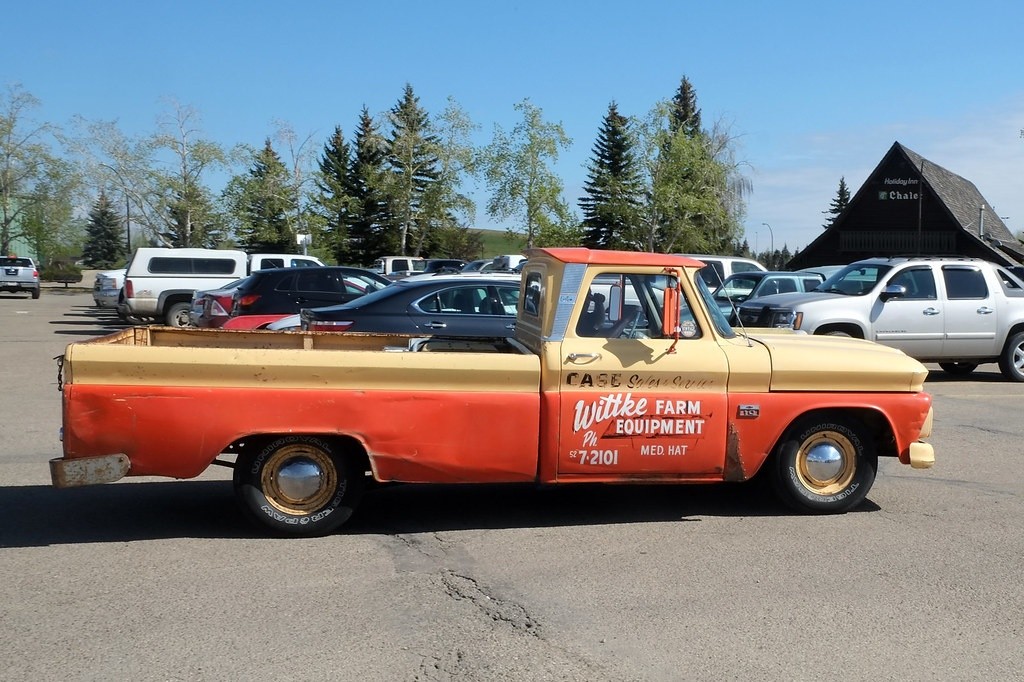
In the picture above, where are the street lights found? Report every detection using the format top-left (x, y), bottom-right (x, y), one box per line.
top-left (762, 223), bottom-right (773, 262)
top-left (98, 162), bottom-right (131, 254)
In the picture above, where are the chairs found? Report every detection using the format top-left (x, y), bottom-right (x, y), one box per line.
top-left (442, 293), bottom-right (498, 314)
top-left (525, 294), bottom-right (538, 316)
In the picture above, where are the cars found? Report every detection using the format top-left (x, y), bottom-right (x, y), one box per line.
top-left (300, 278), bottom-right (616, 338)
top-left (188, 275), bottom-right (365, 328)
top-left (93, 267), bottom-right (153, 326)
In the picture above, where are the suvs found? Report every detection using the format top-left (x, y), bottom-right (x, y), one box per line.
top-left (232, 265), bottom-right (391, 318)
top-left (0, 256), bottom-right (42, 299)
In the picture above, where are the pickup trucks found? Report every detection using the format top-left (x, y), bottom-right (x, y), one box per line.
top-left (729, 254), bottom-right (1024, 384)
top-left (48, 247), bottom-right (936, 539)
top-left (222, 253), bottom-right (879, 339)
top-left (117, 248), bottom-right (326, 328)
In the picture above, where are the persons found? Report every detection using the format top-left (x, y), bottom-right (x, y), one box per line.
top-left (578, 288), bottom-right (605, 335)
